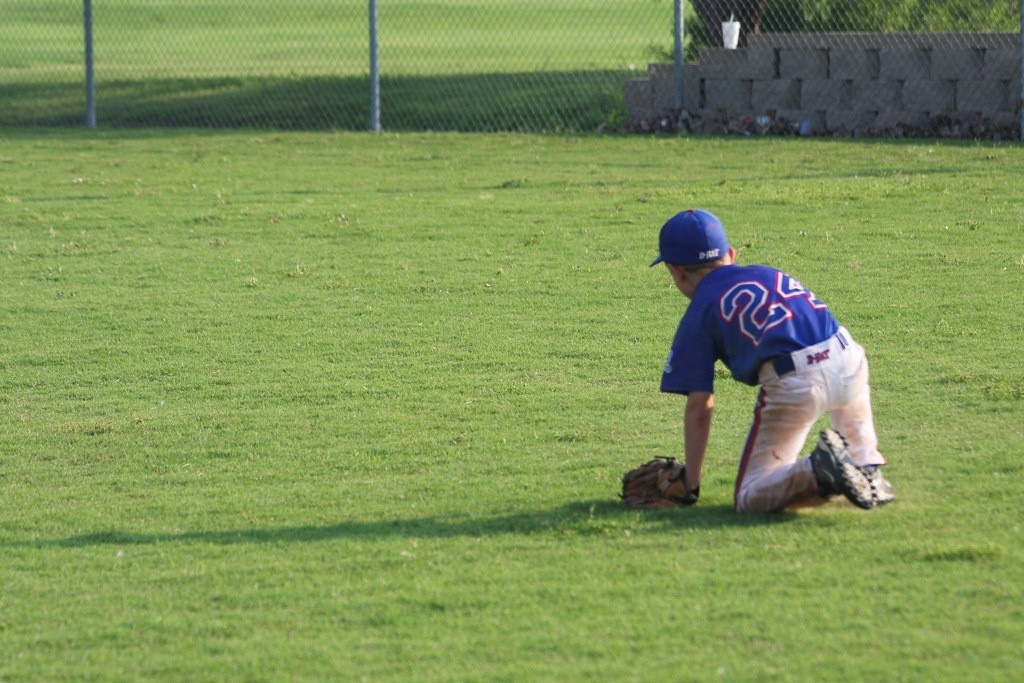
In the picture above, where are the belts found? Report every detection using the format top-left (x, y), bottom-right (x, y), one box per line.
top-left (772, 333), bottom-right (848, 377)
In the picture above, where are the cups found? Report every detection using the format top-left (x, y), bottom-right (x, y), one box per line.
top-left (723, 22), bottom-right (740, 49)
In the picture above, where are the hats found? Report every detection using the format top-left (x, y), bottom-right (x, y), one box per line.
top-left (649, 210), bottom-right (728, 267)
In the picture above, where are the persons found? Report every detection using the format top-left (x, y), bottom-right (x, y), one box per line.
top-left (650, 209), bottom-right (894, 516)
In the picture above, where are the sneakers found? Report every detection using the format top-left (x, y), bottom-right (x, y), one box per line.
top-left (811, 428), bottom-right (878, 510)
top-left (857, 464), bottom-right (894, 503)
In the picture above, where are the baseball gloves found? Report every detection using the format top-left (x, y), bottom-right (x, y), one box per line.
top-left (618, 454), bottom-right (700, 510)
